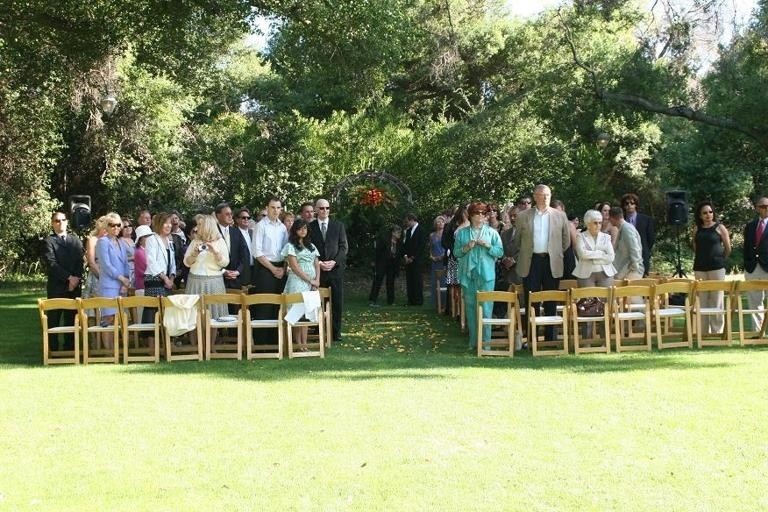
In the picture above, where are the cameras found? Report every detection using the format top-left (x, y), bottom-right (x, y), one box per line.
top-left (198, 243), bottom-right (209, 252)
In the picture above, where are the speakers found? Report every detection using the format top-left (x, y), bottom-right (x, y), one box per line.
top-left (68, 194), bottom-right (91, 228)
top-left (665, 190), bottom-right (689, 227)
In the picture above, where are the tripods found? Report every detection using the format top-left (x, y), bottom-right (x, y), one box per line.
top-left (670, 228), bottom-right (687, 278)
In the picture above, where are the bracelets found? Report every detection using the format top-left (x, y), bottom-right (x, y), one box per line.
top-left (404, 255), bottom-right (407, 257)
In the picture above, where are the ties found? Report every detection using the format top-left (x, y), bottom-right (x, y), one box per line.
top-left (61, 235), bottom-right (65, 245)
top-left (321, 223), bottom-right (327, 241)
top-left (755, 220), bottom-right (765, 246)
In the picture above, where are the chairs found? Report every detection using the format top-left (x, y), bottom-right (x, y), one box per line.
top-left (38, 284), bottom-right (333, 366)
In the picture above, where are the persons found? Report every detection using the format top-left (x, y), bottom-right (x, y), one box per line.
top-left (428, 183), bottom-right (655, 355)
top-left (692, 203), bottom-right (731, 334)
top-left (368, 226), bottom-right (404, 306)
top-left (401, 214), bottom-right (430, 306)
top-left (44, 198), bottom-right (349, 352)
top-left (744, 197), bottom-right (768, 338)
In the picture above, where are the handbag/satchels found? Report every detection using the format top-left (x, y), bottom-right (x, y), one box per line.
top-left (576, 296), bottom-right (605, 317)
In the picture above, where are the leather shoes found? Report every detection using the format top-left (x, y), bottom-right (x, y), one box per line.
top-left (333, 337), bottom-right (342, 341)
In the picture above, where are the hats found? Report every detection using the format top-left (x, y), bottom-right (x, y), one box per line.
top-left (134, 225), bottom-right (156, 244)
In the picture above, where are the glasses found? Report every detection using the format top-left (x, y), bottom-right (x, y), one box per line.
top-left (594, 222), bottom-right (602, 224)
top-left (108, 223), bottom-right (121, 227)
top-left (225, 212), bottom-right (233, 216)
top-left (238, 216), bottom-right (251, 219)
top-left (703, 210), bottom-right (713, 214)
top-left (55, 219), bottom-right (66, 223)
top-left (759, 205), bottom-right (768, 208)
top-left (488, 209), bottom-right (497, 212)
top-left (192, 230), bottom-right (197, 233)
top-left (124, 225), bottom-right (133, 228)
top-left (475, 211), bottom-right (487, 215)
top-left (625, 201), bottom-right (635, 205)
top-left (520, 201), bottom-right (532, 205)
top-left (316, 207), bottom-right (329, 210)
